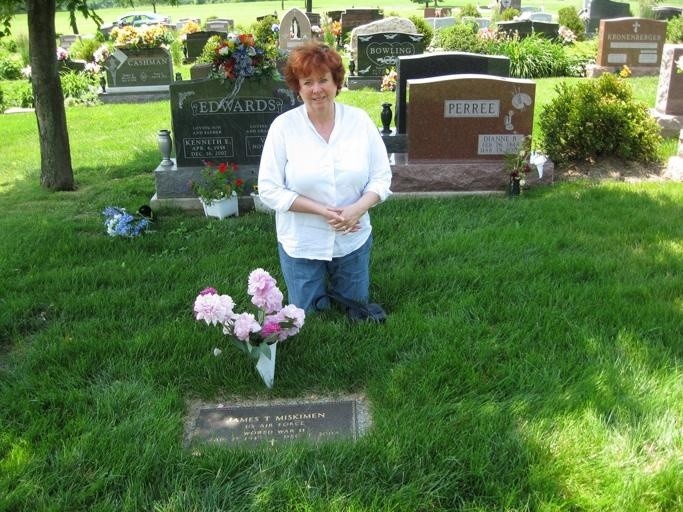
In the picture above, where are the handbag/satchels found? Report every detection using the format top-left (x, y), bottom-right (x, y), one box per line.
top-left (312, 294), bottom-right (389, 322)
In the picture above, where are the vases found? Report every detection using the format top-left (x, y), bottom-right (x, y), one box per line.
top-left (156, 130), bottom-right (174, 167)
top-left (250, 192), bottom-right (273, 215)
top-left (245, 338), bottom-right (278, 389)
top-left (510, 176), bottom-right (521, 196)
top-left (196, 191), bottom-right (239, 221)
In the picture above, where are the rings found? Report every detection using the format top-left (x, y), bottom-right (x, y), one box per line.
top-left (342, 225), bottom-right (346, 230)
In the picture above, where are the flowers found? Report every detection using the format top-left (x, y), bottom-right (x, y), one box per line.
top-left (379, 67), bottom-right (397, 92)
top-left (102, 207), bottom-right (148, 237)
top-left (619, 64), bottom-right (631, 78)
top-left (193, 266), bottom-right (305, 364)
top-left (187, 158), bottom-right (243, 207)
top-left (201, 35), bottom-right (289, 100)
top-left (109, 24), bottom-right (174, 50)
top-left (502, 134), bottom-right (534, 186)
top-left (251, 180), bottom-right (258, 195)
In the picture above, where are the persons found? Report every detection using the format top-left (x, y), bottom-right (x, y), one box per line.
top-left (256, 43), bottom-right (392, 317)
